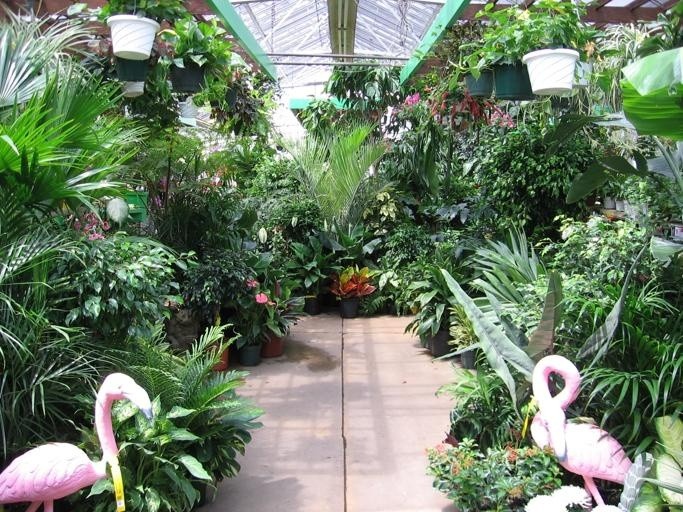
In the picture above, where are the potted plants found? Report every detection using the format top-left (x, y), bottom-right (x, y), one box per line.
top-left (280, 57), bottom-right (405, 319)
top-left (2, 1), bottom-right (292, 512)
top-left (394, 0), bottom-right (682, 512)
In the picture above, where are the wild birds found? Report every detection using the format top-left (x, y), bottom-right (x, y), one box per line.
top-left (0, 371), bottom-right (151, 512)
top-left (529, 353), bottom-right (633, 510)
